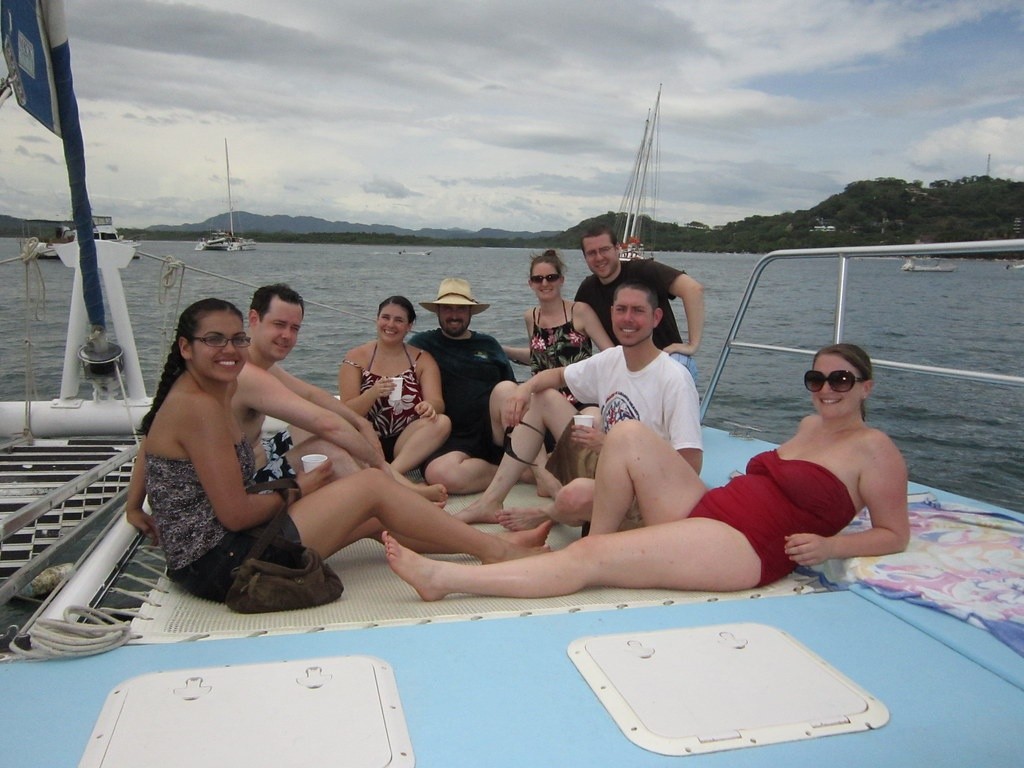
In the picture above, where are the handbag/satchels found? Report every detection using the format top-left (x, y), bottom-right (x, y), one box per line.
top-left (224, 547), bottom-right (344, 614)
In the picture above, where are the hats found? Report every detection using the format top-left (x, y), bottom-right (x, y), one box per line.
top-left (418, 278), bottom-right (490, 315)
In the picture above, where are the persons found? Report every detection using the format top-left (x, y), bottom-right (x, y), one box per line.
top-left (229, 284), bottom-right (449, 510)
top-left (126, 298), bottom-right (554, 603)
top-left (380, 345), bottom-right (911, 600)
top-left (500, 252), bottom-right (616, 454)
top-left (406, 278), bottom-right (565, 500)
top-left (451, 277), bottom-right (703, 533)
top-left (574, 223), bottom-right (705, 383)
top-left (339, 295), bottom-right (451, 474)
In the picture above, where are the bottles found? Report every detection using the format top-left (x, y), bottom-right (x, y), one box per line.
top-left (23, 563), bottom-right (75, 597)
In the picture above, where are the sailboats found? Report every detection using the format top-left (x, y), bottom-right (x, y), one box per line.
top-left (610, 84), bottom-right (663, 263)
top-left (200, 137), bottom-right (257, 250)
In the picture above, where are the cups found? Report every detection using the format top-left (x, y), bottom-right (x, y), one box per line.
top-left (389, 378), bottom-right (403, 400)
top-left (301, 454), bottom-right (327, 473)
top-left (574, 415), bottom-right (594, 433)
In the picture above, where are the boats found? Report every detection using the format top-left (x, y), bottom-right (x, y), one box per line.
top-left (901, 260), bottom-right (958, 272)
top-left (1005, 263), bottom-right (1023, 269)
top-left (0, 232), bottom-right (1023, 766)
top-left (396, 250), bottom-right (432, 255)
top-left (31, 216), bottom-right (142, 260)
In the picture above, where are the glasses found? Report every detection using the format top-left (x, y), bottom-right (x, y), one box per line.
top-left (530, 274), bottom-right (560, 283)
top-left (189, 335), bottom-right (251, 348)
top-left (804, 370), bottom-right (865, 392)
top-left (503, 420), bottom-right (547, 467)
